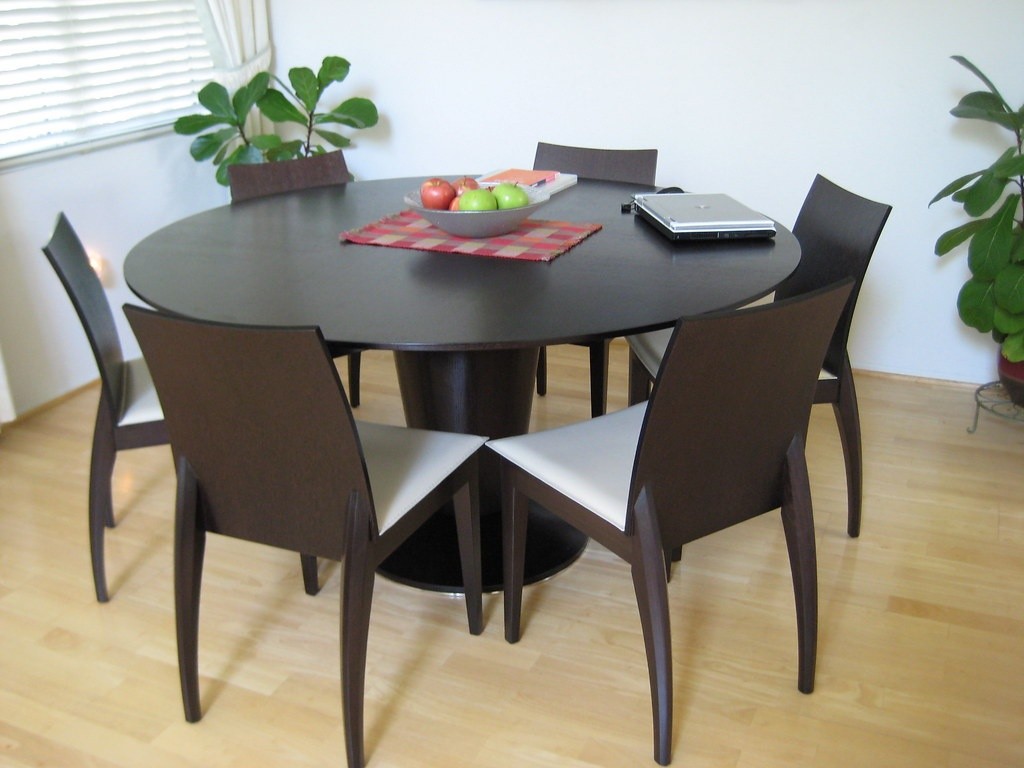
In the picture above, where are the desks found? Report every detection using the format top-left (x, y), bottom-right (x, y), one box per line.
top-left (123, 174), bottom-right (802, 593)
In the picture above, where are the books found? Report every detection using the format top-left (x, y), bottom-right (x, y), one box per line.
top-left (477, 171), bottom-right (578, 196)
top-left (480, 167), bottom-right (559, 188)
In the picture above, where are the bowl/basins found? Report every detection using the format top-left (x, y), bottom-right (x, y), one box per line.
top-left (405, 181), bottom-right (551, 238)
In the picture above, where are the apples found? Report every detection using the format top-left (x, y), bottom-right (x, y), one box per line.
top-left (421, 178), bottom-right (529, 210)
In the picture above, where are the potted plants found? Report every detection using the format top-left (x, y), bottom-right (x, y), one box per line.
top-left (928, 52), bottom-right (1024, 407)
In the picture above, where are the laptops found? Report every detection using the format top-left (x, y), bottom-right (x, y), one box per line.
top-left (635, 192), bottom-right (776, 242)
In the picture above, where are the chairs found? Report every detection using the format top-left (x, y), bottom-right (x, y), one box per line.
top-left (122, 302), bottom-right (490, 768)
top-left (623, 172), bottom-right (893, 538)
top-left (532, 141), bottom-right (658, 418)
top-left (484, 275), bottom-right (860, 766)
top-left (41, 210), bottom-right (319, 603)
top-left (227, 149), bottom-right (361, 409)
